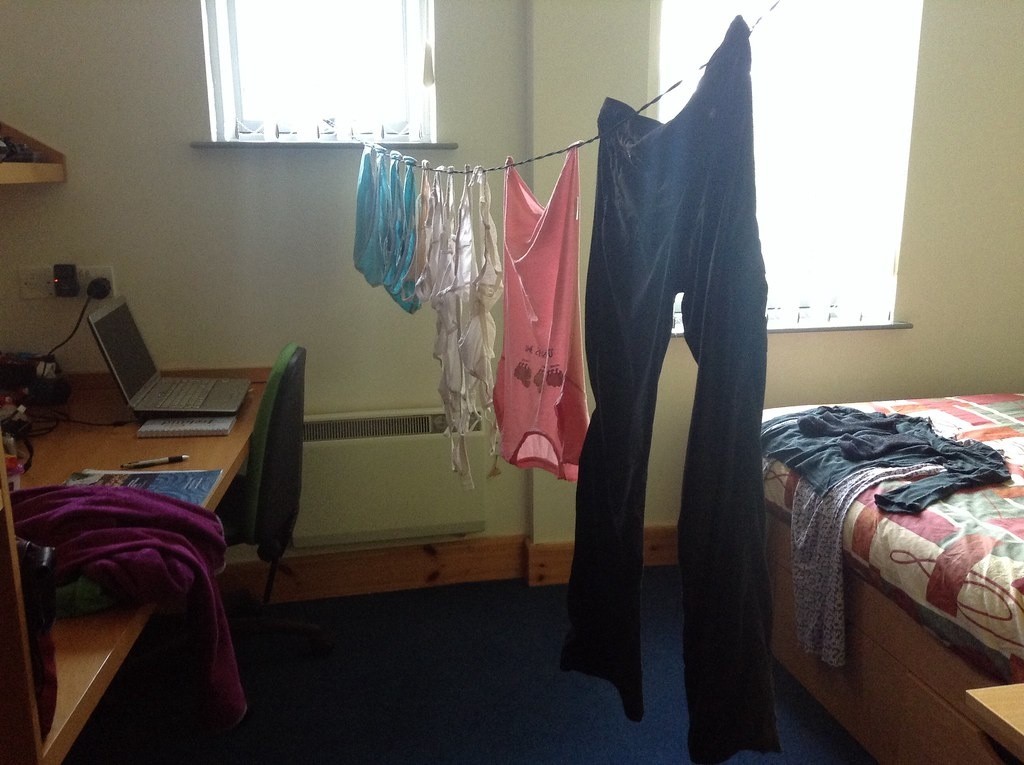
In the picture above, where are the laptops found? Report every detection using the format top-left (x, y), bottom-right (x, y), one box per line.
top-left (86, 294), bottom-right (251, 416)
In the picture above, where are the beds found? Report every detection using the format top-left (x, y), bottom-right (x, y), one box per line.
top-left (759, 393), bottom-right (1024, 765)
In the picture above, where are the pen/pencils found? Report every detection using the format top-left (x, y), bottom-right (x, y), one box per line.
top-left (121, 454), bottom-right (189, 468)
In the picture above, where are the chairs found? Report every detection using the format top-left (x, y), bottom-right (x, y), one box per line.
top-left (209, 344), bottom-right (307, 618)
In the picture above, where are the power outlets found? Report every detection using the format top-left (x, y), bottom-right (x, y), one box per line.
top-left (18, 263), bottom-right (117, 303)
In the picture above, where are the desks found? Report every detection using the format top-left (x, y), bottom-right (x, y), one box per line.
top-left (0, 366), bottom-right (272, 765)
